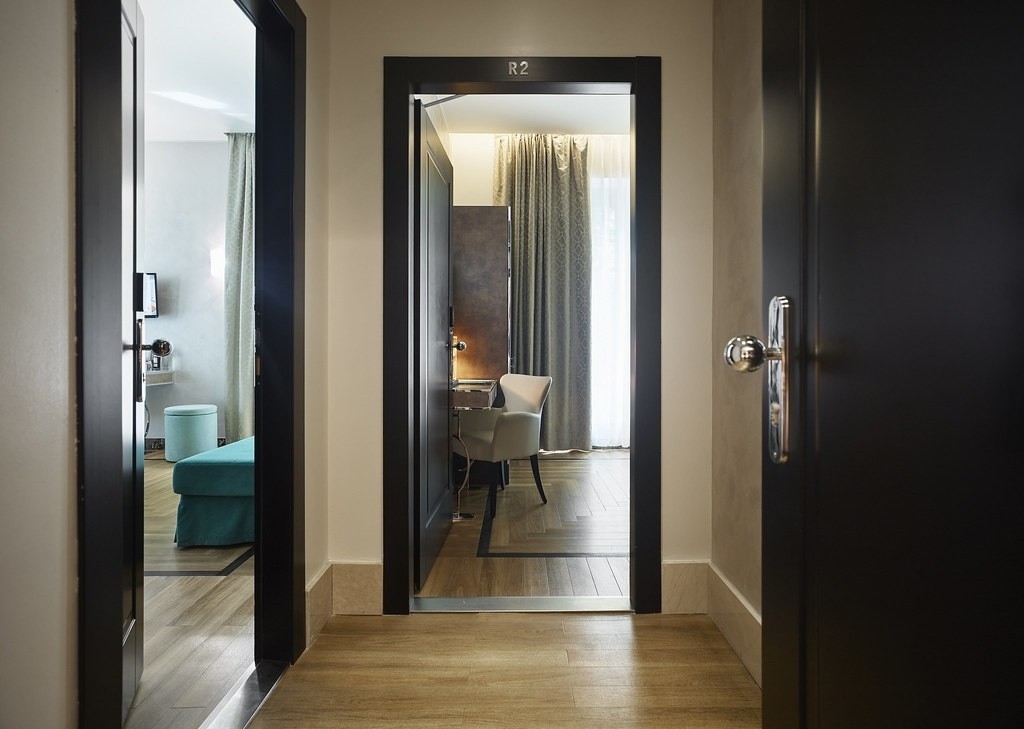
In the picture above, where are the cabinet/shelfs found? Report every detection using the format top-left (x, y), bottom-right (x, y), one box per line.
top-left (455, 205), bottom-right (512, 392)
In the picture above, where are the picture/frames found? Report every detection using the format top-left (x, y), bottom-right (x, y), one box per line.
top-left (142, 273), bottom-right (158, 318)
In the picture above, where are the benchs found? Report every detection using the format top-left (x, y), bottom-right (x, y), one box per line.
top-left (174, 435), bottom-right (255, 548)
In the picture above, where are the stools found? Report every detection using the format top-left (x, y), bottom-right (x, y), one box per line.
top-left (164, 404), bottom-right (217, 462)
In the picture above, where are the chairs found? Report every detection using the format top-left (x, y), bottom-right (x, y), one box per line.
top-left (451, 374), bottom-right (552, 518)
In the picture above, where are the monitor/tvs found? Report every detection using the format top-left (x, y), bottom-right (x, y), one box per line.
top-left (136, 273), bottom-right (158, 319)
